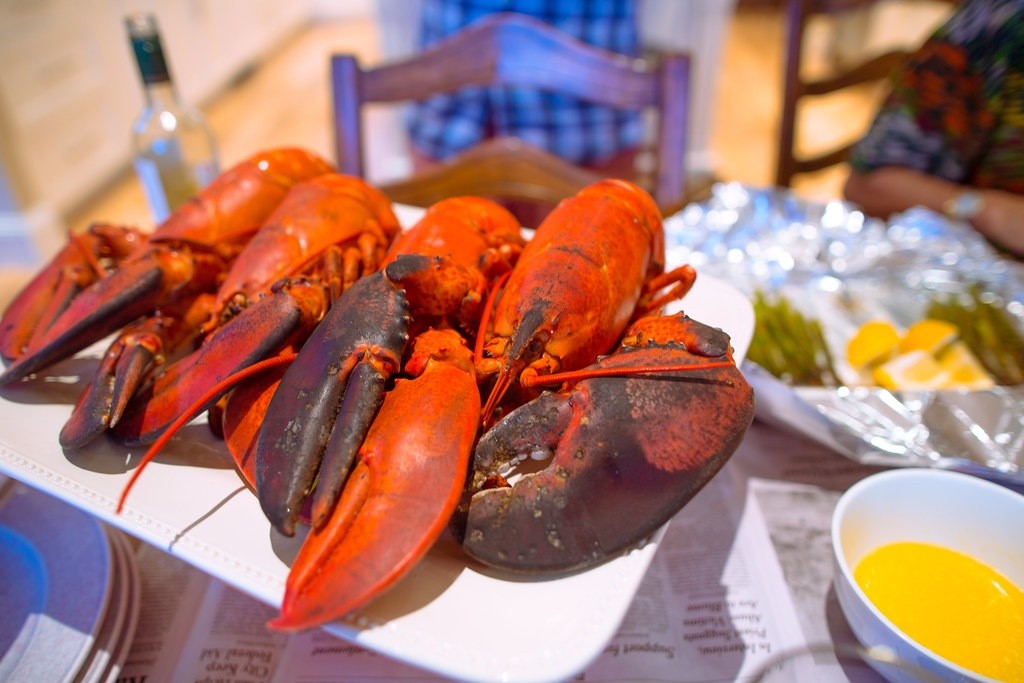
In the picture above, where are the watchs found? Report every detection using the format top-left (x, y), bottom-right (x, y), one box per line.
top-left (944, 185), bottom-right (985, 223)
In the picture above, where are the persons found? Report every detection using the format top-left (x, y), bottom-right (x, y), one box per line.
top-left (843, 1), bottom-right (1024, 256)
top-left (408, 1), bottom-right (640, 229)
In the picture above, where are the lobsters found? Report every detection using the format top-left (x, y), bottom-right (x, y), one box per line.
top-left (0, 147), bottom-right (757, 634)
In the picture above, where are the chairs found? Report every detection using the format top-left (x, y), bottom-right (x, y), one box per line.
top-left (777, 0), bottom-right (973, 189)
top-left (331, 11), bottom-right (690, 224)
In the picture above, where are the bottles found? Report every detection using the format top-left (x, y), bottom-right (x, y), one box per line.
top-left (124, 12), bottom-right (223, 222)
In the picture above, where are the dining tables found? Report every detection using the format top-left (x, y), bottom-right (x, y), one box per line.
top-left (0, 202), bottom-right (1024, 682)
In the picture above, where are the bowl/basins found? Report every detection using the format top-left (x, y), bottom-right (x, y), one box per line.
top-left (830, 468), bottom-right (1024, 683)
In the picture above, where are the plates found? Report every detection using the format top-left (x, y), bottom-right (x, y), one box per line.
top-left (0, 485), bottom-right (142, 683)
top-left (0, 198), bottom-right (755, 683)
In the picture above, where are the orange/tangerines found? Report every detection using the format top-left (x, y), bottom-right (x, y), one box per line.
top-left (849, 319), bottom-right (996, 394)
